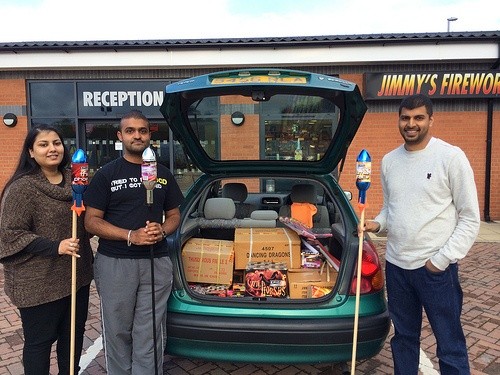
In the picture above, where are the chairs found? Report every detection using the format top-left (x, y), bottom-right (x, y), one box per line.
top-left (279, 185), bottom-right (330, 227)
top-left (220, 184), bottom-right (255, 216)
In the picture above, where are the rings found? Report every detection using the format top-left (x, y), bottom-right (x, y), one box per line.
top-left (70, 243), bottom-right (73, 246)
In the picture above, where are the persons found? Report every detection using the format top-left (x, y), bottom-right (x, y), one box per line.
top-left (0, 124), bottom-right (94, 375)
top-left (83, 112), bottom-right (185, 375)
top-left (357, 93), bottom-right (480, 374)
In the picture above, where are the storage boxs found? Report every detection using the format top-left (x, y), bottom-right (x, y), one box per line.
top-left (287, 266), bottom-right (339, 299)
top-left (183, 238), bottom-right (233, 286)
top-left (234, 227), bottom-right (302, 271)
top-left (244, 261), bottom-right (289, 298)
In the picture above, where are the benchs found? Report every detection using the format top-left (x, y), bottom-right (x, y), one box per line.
top-left (200, 198), bottom-right (278, 238)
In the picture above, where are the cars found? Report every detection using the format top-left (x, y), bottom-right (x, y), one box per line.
top-left (159, 68), bottom-right (392, 365)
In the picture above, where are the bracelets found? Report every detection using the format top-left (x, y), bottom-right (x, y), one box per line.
top-left (127, 230), bottom-right (131, 246)
top-left (163, 231), bottom-right (167, 236)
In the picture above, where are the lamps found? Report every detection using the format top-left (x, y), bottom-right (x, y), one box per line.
top-left (446, 17), bottom-right (458, 31)
